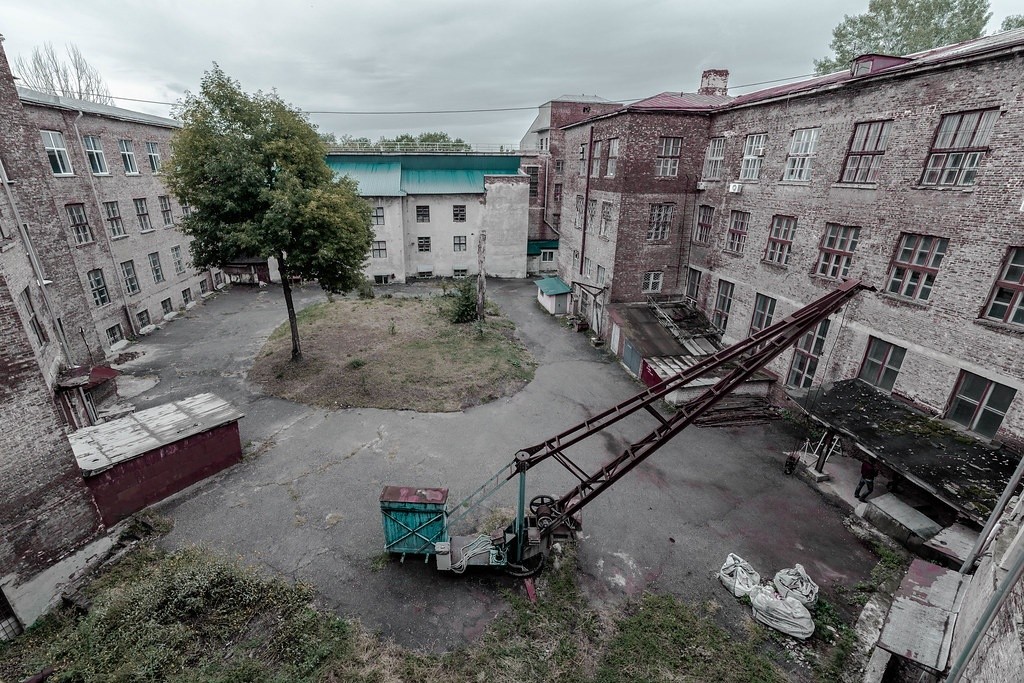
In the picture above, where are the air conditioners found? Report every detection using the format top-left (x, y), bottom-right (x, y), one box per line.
top-left (579, 146), bottom-right (584, 152)
top-left (579, 154), bottom-right (584, 158)
top-left (729, 183), bottom-right (743, 193)
top-left (697, 181), bottom-right (707, 190)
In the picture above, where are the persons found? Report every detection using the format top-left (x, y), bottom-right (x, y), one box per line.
top-left (855, 455), bottom-right (878, 503)
top-left (886, 471), bottom-right (905, 492)
